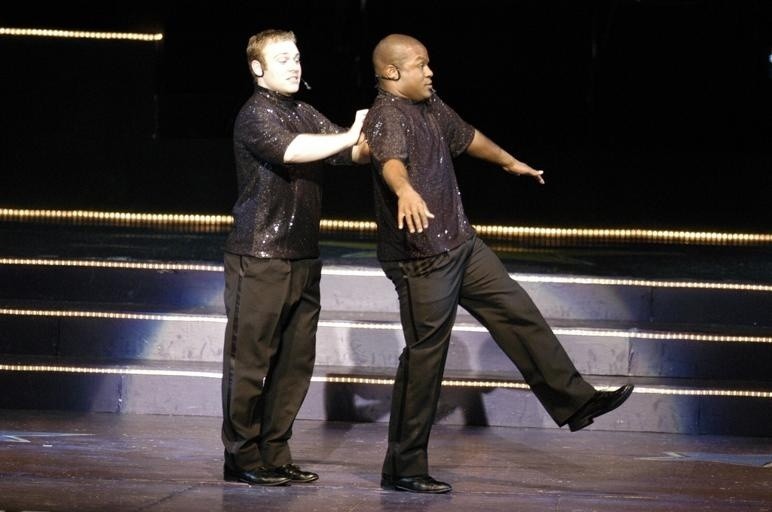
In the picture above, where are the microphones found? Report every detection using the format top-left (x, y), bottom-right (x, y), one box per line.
top-left (301, 78), bottom-right (312, 90)
top-left (432, 88), bottom-right (436, 93)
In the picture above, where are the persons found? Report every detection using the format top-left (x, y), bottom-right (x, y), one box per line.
top-left (361, 32), bottom-right (634, 493)
top-left (218, 28), bottom-right (377, 489)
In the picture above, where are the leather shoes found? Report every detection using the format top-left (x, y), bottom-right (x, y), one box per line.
top-left (280, 464), bottom-right (321, 483)
top-left (380, 472), bottom-right (455, 496)
top-left (567, 382), bottom-right (636, 436)
top-left (221, 465), bottom-right (294, 488)
